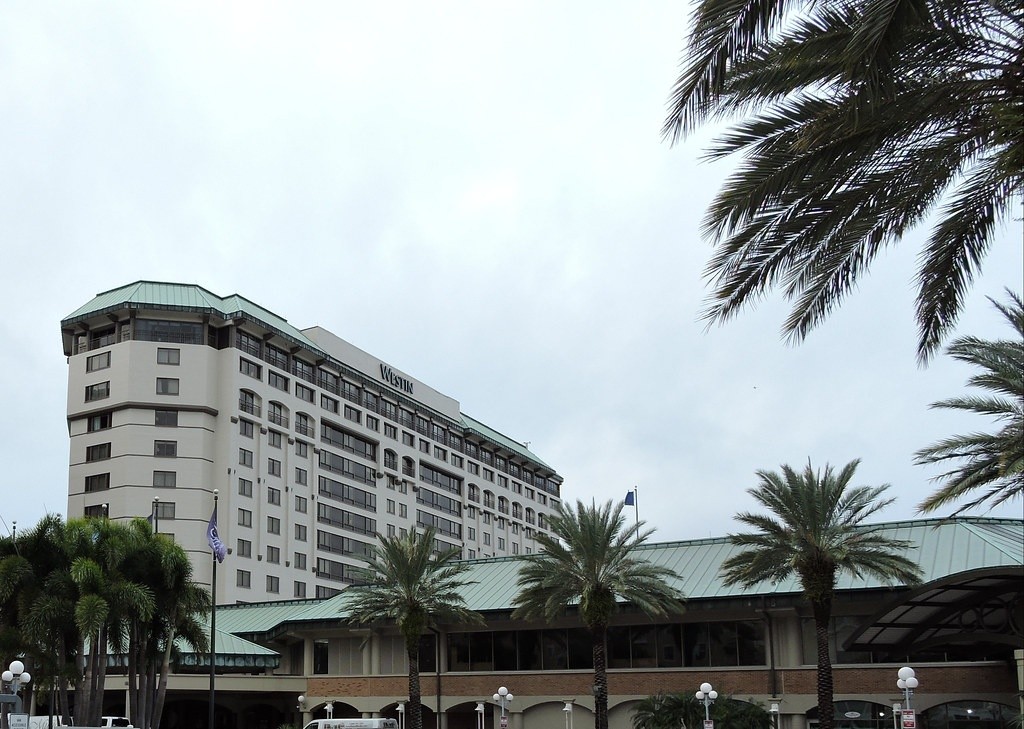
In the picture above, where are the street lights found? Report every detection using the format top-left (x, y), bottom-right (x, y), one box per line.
top-left (0, 660), bottom-right (32, 695)
top-left (896, 666), bottom-right (919, 710)
top-left (492, 686), bottom-right (514, 716)
top-left (695, 682), bottom-right (718, 720)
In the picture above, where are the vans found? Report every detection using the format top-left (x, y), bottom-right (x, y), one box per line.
top-left (69, 716), bottom-right (134, 729)
top-left (302, 717), bottom-right (399, 729)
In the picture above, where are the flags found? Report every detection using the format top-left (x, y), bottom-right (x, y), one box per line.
top-left (625, 491), bottom-right (634, 506)
top-left (206, 508), bottom-right (228, 564)
